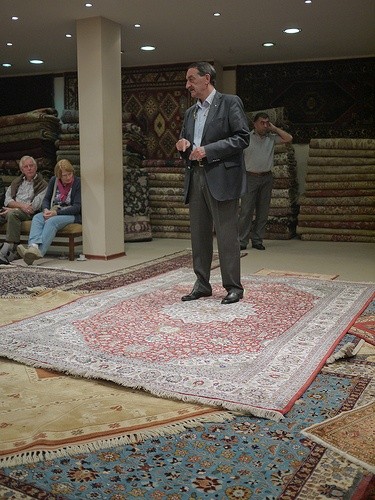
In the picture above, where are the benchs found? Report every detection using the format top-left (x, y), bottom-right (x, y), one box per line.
top-left (0, 183), bottom-right (82, 261)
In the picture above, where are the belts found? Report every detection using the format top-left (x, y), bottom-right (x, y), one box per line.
top-left (247, 170), bottom-right (272, 176)
top-left (192, 160), bottom-right (204, 167)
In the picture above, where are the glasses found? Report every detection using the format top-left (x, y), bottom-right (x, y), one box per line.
top-left (260, 121), bottom-right (269, 125)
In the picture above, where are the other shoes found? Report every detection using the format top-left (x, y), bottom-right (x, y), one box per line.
top-left (0, 242), bottom-right (15, 264)
top-left (16, 245), bottom-right (39, 259)
top-left (23, 252), bottom-right (36, 265)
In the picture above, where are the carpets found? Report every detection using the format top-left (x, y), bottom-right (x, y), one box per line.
top-left (1, 266), bottom-right (375, 423)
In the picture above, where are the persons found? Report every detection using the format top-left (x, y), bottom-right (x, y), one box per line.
top-left (0, 155), bottom-right (49, 264)
top-left (241, 113), bottom-right (293, 251)
top-left (23, 159), bottom-right (81, 265)
top-left (175, 62), bottom-right (249, 306)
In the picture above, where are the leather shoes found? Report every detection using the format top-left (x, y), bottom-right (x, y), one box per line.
top-left (221, 291), bottom-right (243, 304)
top-left (252, 244), bottom-right (265, 250)
top-left (181, 288), bottom-right (212, 301)
top-left (240, 246), bottom-right (247, 250)
